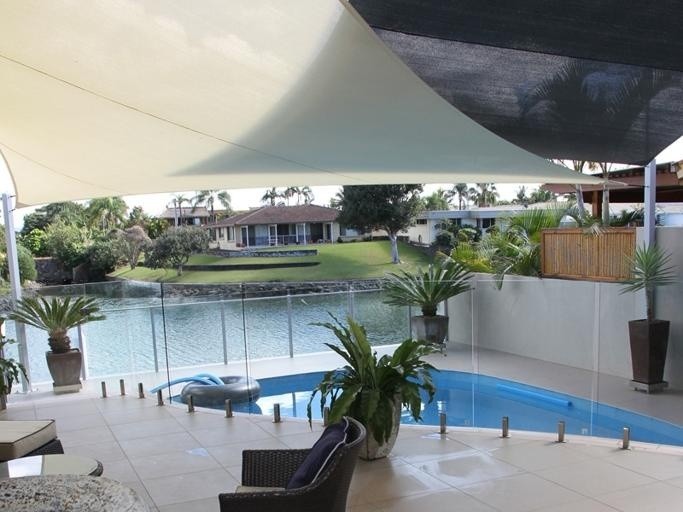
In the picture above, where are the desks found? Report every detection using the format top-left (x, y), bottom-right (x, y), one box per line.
top-left (0, 454), bottom-right (103, 483)
top-left (0, 474), bottom-right (151, 512)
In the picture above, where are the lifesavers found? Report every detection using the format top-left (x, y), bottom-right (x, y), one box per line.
top-left (181, 377), bottom-right (260, 405)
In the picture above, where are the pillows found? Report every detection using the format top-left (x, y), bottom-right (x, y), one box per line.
top-left (285, 416), bottom-right (350, 488)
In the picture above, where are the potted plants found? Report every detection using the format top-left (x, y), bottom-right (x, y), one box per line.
top-left (380, 260), bottom-right (476, 351)
top-left (617, 240), bottom-right (679, 394)
top-left (307, 309), bottom-right (440, 462)
top-left (9, 295), bottom-right (107, 394)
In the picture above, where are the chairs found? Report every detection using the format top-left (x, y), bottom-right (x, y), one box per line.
top-left (217, 416), bottom-right (368, 512)
top-left (0, 419), bottom-right (65, 460)
